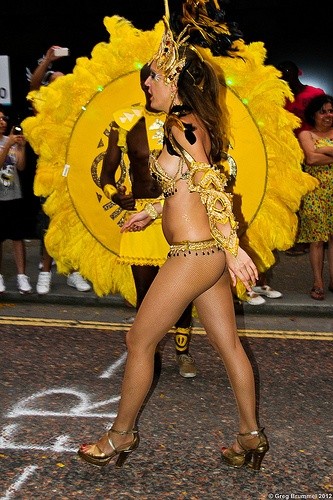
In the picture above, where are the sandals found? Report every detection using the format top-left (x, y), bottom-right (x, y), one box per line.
top-left (310, 284), bottom-right (325, 300)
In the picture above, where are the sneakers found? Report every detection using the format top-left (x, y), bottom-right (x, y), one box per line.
top-left (0, 274), bottom-right (5, 292)
top-left (36, 270), bottom-right (51, 294)
top-left (174, 353), bottom-right (197, 378)
top-left (67, 272), bottom-right (92, 291)
top-left (17, 274), bottom-right (31, 291)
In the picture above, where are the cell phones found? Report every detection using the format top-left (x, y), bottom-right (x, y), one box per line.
top-left (13, 127), bottom-right (22, 135)
top-left (54, 47), bottom-right (69, 56)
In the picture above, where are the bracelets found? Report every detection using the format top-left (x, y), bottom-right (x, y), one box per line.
top-left (143, 202), bottom-right (160, 220)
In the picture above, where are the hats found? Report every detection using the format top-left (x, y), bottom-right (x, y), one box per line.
top-left (279, 61), bottom-right (299, 72)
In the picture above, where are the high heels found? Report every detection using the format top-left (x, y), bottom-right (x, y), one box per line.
top-left (78, 429), bottom-right (140, 469)
top-left (219, 427), bottom-right (269, 470)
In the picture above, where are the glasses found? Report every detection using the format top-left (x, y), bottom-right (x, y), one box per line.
top-left (0, 116), bottom-right (9, 122)
top-left (283, 72), bottom-right (293, 78)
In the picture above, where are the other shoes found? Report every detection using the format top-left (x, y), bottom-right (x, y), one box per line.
top-left (127, 312), bottom-right (137, 323)
top-left (285, 243), bottom-right (310, 256)
top-left (253, 284), bottom-right (283, 299)
top-left (239, 292), bottom-right (265, 305)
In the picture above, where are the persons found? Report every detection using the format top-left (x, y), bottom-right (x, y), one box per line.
top-left (279, 62), bottom-right (325, 138)
top-left (131, 264), bottom-right (197, 377)
top-left (239, 271), bottom-right (283, 304)
top-left (0, 111), bottom-right (33, 293)
top-left (296, 94), bottom-right (333, 301)
top-left (76, 0), bottom-right (270, 474)
top-left (18, 45), bottom-right (92, 294)
